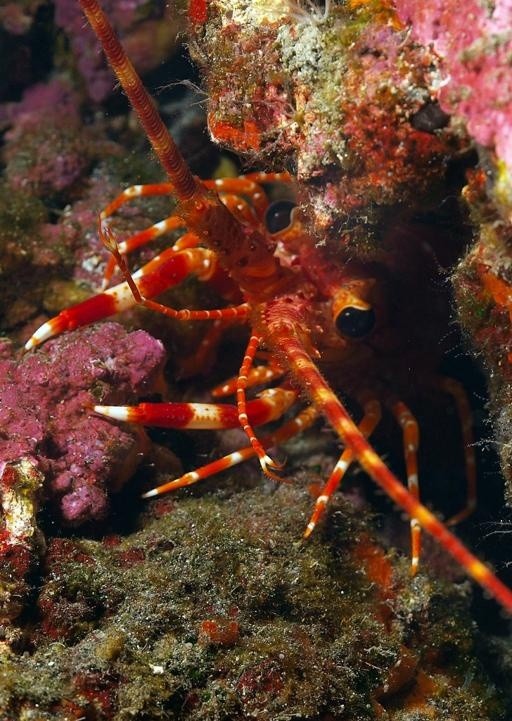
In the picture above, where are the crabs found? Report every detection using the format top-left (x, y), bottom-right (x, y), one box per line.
top-left (19, 0), bottom-right (512, 615)
